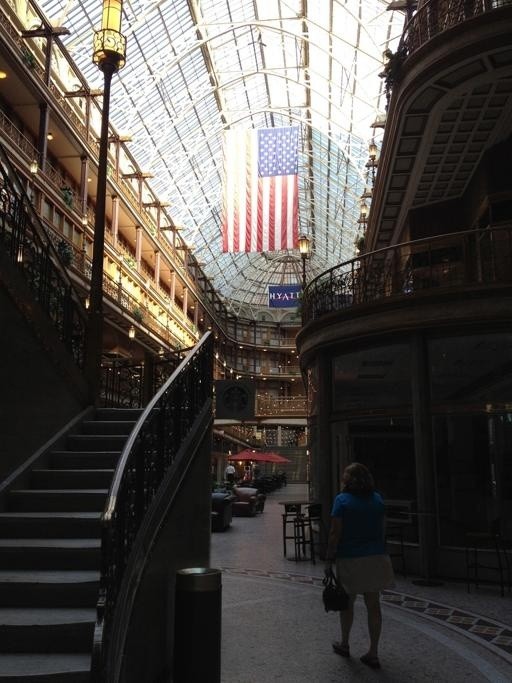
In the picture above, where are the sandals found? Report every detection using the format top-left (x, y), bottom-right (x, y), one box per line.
top-left (359, 652), bottom-right (380, 669)
top-left (332, 641), bottom-right (349, 657)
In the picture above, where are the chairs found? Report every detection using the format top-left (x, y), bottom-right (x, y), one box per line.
top-left (212, 487), bottom-right (266, 532)
top-left (279, 500), bottom-right (328, 565)
top-left (464, 516), bottom-right (511, 597)
top-left (385, 523), bottom-right (406, 577)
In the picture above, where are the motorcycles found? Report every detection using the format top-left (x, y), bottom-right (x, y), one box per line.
top-left (273, 471), bottom-right (288, 488)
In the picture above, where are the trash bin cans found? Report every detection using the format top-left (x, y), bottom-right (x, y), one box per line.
top-left (174, 567), bottom-right (222, 683)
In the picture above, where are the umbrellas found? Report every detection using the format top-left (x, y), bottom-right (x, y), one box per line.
top-left (262, 451), bottom-right (292, 464)
top-left (227, 447), bottom-right (275, 461)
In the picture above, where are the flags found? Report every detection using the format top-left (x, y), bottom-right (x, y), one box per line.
top-left (223, 123), bottom-right (301, 253)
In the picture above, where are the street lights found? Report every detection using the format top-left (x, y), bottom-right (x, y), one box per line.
top-left (82, 0), bottom-right (126, 405)
top-left (296, 235), bottom-right (312, 318)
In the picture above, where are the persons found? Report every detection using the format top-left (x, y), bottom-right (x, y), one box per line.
top-left (225, 462), bottom-right (235, 480)
top-left (322, 462), bottom-right (395, 667)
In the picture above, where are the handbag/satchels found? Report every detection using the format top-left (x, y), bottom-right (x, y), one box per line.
top-left (322, 572), bottom-right (349, 613)
